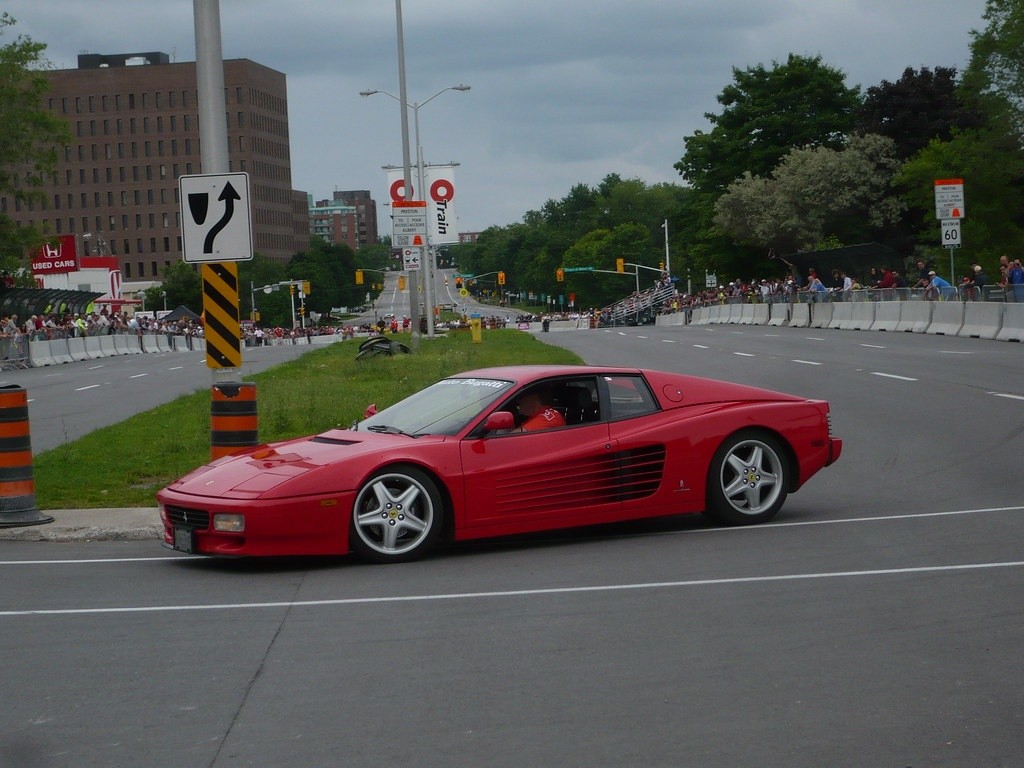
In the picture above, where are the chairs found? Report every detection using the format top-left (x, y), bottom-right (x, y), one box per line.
top-left (557, 386), bottom-right (601, 428)
top-left (499, 401), bottom-right (521, 427)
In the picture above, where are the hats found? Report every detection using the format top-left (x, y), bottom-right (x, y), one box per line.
top-left (390, 314), bottom-right (394, 316)
top-left (74, 313), bottom-right (79, 317)
top-left (928, 271), bottom-right (936, 275)
top-left (87, 315), bottom-right (92, 321)
top-left (787, 280), bottom-right (793, 284)
top-left (403, 315), bottom-right (406, 318)
top-left (719, 286), bottom-right (724, 289)
top-left (729, 282), bottom-right (734, 286)
top-left (761, 279), bottom-right (767, 283)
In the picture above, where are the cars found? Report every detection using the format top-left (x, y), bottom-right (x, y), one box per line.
top-left (321, 315), bottom-right (341, 322)
top-left (384, 310), bottom-right (395, 319)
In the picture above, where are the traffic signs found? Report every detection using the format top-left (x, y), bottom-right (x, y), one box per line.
top-left (402, 247), bottom-right (421, 271)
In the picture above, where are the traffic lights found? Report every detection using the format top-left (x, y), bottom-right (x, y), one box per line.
top-left (658, 262), bottom-right (664, 270)
top-left (301, 306), bottom-right (305, 316)
top-left (557, 268), bottom-right (564, 281)
top-left (457, 277), bottom-right (461, 282)
top-left (455, 276), bottom-right (463, 289)
top-left (498, 273), bottom-right (505, 285)
top-left (290, 286), bottom-right (295, 295)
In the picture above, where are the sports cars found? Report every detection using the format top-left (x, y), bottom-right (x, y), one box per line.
top-left (155, 364), bottom-right (842, 564)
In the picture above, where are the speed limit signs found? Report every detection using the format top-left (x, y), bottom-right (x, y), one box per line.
top-left (941, 220), bottom-right (961, 250)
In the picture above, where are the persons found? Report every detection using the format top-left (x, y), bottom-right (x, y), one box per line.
top-left (377, 314), bottom-right (412, 335)
top-left (239, 323), bottom-right (374, 347)
top-left (516, 272), bottom-right (670, 326)
top-left (496, 385), bottom-right (566, 435)
top-left (658, 256), bottom-right (1024, 327)
top-left (1, 305), bottom-right (205, 341)
top-left (435, 314), bottom-right (510, 329)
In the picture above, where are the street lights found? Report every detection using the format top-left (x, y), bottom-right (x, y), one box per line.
top-left (360, 84), bottom-right (471, 340)
top-left (661, 218), bottom-right (670, 276)
